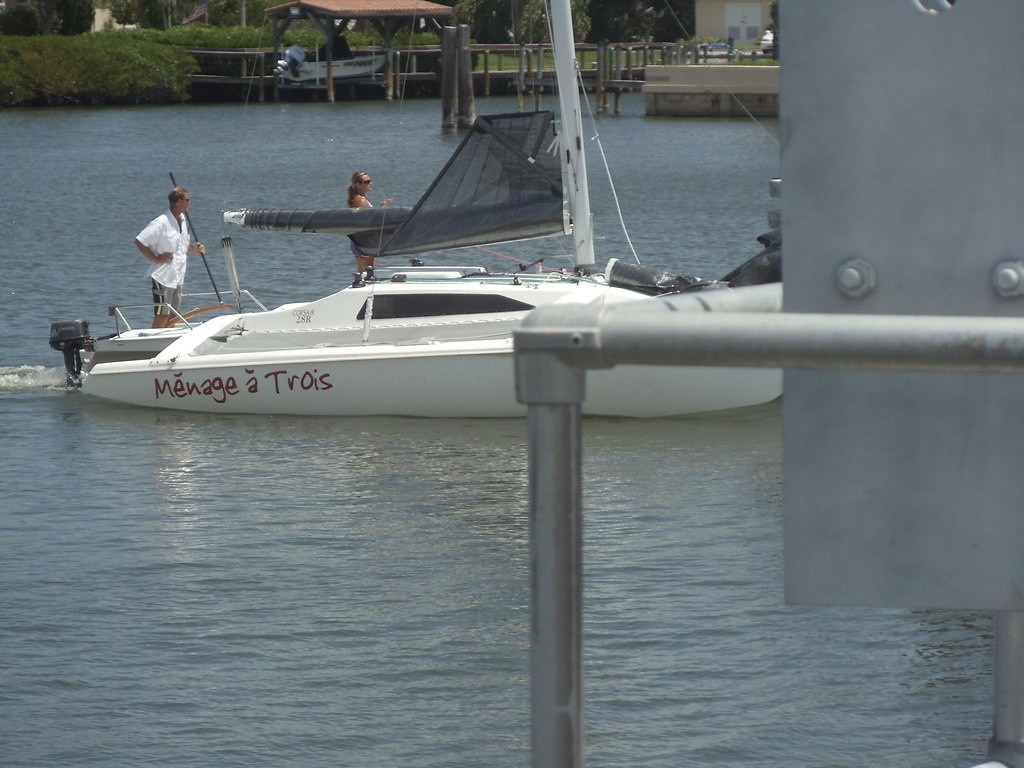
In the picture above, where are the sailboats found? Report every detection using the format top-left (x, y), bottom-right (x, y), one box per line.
top-left (48, 0), bottom-right (783, 419)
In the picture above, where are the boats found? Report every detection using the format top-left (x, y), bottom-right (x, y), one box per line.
top-left (273, 36), bottom-right (387, 82)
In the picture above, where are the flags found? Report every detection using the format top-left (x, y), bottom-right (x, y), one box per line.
top-left (181, 0), bottom-right (207, 24)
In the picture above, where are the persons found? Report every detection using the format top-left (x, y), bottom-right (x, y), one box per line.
top-left (135, 187), bottom-right (205, 328)
top-left (346, 171), bottom-right (393, 273)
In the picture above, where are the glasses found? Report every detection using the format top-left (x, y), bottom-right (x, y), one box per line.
top-left (355, 180), bottom-right (370, 184)
top-left (185, 198), bottom-right (190, 202)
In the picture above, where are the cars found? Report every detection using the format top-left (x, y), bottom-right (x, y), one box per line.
top-left (761, 25), bottom-right (775, 54)
top-left (699, 42), bottom-right (734, 51)
